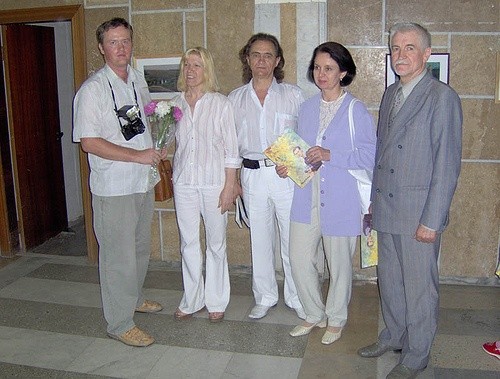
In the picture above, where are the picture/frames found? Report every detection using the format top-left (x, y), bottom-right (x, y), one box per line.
top-left (385, 53), bottom-right (450, 90)
top-left (132, 52), bottom-right (182, 100)
top-left (493, 51), bottom-right (500, 104)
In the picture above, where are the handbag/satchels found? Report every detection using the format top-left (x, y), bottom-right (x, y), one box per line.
top-left (348, 97), bottom-right (372, 214)
top-left (154, 159), bottom-right (174, 202)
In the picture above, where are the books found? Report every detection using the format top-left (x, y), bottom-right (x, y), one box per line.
top-left (360, 214), bottom-right (379, 268)
top-left (263, 127), bottom-right (325, 188)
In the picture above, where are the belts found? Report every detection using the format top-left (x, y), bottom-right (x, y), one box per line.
top-left (258, 158), bottom-right (275, 168)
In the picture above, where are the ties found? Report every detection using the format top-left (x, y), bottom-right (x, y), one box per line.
top-left (387, 87), bottom-right (403, 135)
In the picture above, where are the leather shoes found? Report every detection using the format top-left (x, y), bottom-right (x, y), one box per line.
top-left (248, 304), bottom-right (278, 318)
top-left (358, 342), bottom-right (404, 357)
top-left (386, 363), bottom-right (427, 379)
top-left (321, 326), bottom-right (343, 345)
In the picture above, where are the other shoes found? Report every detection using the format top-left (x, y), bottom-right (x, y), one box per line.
top-left (174, 305), bottom-right (206, 321)
top-left (107, 326), bottom-right (156, 346)
top-left (210, 312), bottom-right (224, 323)
top-left (289, 318), bottom-right (328, 336)
top-left (135, 298), bottom-right (162, 313)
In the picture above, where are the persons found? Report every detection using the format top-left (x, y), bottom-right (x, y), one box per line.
top-left (72, 18), bottom-right (169, 347)
top-left (481, 340), bottom-right (500, 360)
top-left (275, 42), bottom-right (377, 346)
top-left (228, 33), bottom-right (307, 319)
top-left (357, 22), bottom-right (463, 378)
top-left (168, 47), bottom-right (244, 322)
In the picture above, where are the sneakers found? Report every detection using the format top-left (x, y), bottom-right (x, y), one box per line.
top-left (482, 341), bottom-right (500, 359)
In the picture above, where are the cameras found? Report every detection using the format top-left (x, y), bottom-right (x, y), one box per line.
top-left (122, 118), bottom-right (146, 141)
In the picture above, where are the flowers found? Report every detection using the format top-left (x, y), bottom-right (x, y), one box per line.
top-left (144, 100), bottom-right (182, 152)
